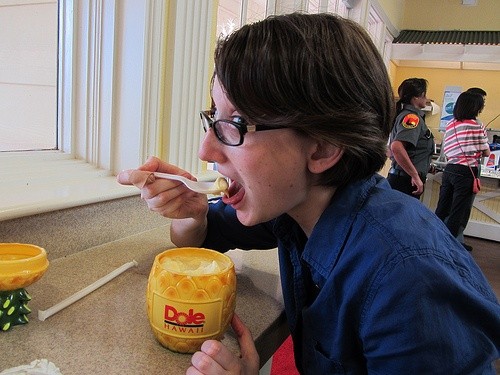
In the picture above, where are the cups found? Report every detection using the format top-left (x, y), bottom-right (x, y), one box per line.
top-left (147, 246), bottom-right (236, 354)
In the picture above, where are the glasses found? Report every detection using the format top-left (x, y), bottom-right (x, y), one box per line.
top-left (200, 110), bottom-right (297, 146)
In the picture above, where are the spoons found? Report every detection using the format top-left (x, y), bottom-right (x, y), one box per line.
top-left (154, 171), bottom-right (221, 194)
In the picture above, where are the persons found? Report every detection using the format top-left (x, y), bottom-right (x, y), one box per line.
top-left (385, 77), bottom-right (491, 253)
top-left (115, 11), bottom-right (500, 375)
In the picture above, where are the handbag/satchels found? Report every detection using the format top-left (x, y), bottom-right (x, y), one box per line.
top-left (472, 177), bottom-right (480, 194)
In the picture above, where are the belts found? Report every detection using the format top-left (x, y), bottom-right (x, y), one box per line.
top-left (389, 168), bottom-right (426, 180)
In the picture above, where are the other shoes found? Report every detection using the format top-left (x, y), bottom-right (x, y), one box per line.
top-left (461, 243), bottom-right (472, 251)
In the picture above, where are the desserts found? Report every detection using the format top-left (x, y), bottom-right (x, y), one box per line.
top-left (214, 176), bottom-right (230, 199)
top-left (146, 248), bottom-right (236, 353)
